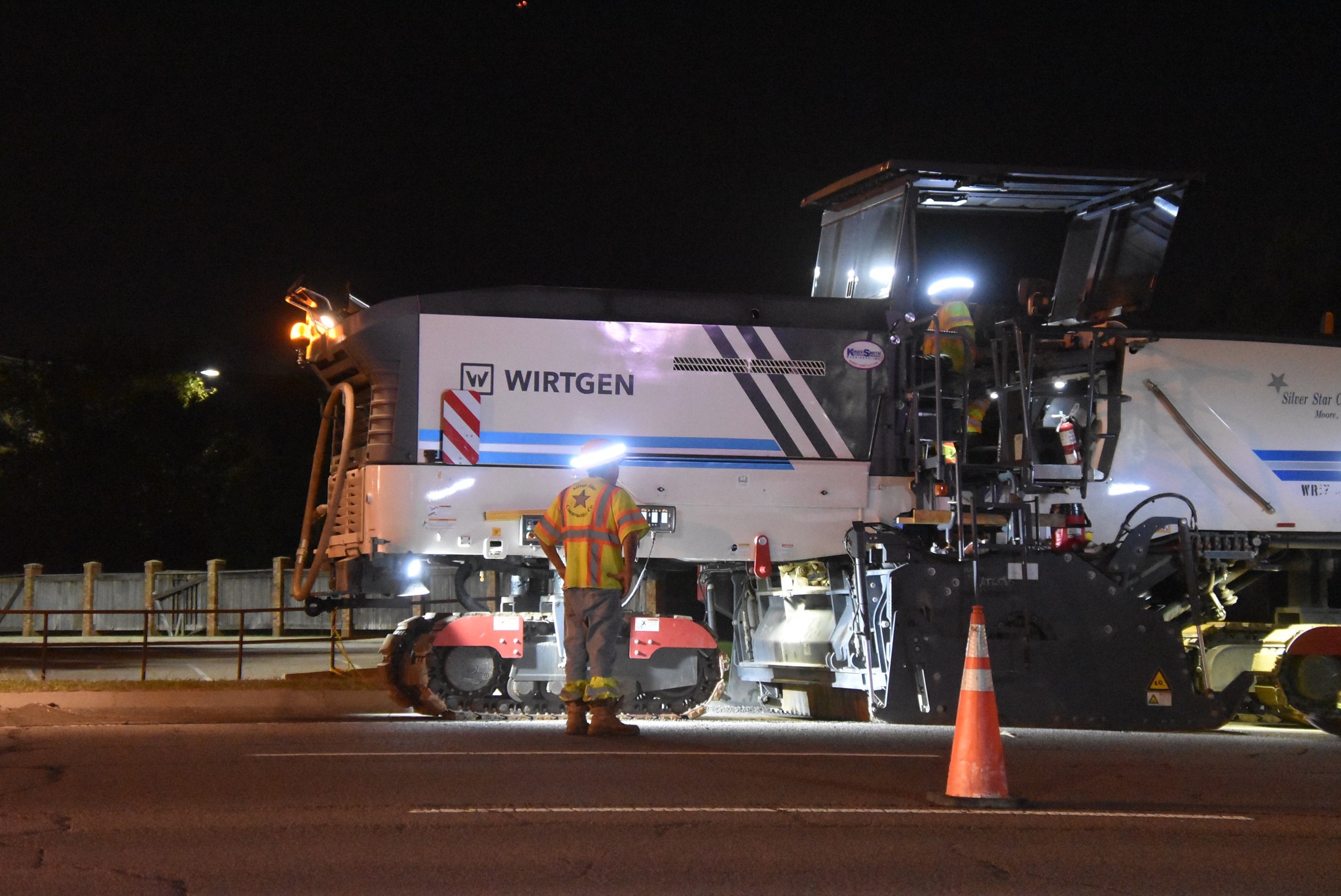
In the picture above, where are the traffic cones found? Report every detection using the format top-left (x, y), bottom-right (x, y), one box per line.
top-left (922, 605), bottom-right (1030, 809)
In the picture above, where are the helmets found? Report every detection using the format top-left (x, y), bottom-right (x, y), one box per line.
top-left (569, 438), bottom-right (626, 469)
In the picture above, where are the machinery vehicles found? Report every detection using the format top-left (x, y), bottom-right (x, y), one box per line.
top-left (733, 150), bottom-right (1341, 733)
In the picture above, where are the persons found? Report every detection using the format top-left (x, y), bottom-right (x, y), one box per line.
top-left (536, 439), bottom-right (643, 735)
top-left (922, 277), bottom-right (993, 462)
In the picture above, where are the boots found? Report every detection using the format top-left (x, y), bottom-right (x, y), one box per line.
top-left (559, 688), bottom-right (590, 735)
top-left (583, 684), bottom-right (641, 737)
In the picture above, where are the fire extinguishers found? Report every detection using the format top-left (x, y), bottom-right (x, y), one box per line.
top-left (1051, 413), bottom-right (1083, 465)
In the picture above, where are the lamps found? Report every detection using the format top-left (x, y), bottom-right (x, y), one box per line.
top-left (920, 194), bottom-right (968, 206)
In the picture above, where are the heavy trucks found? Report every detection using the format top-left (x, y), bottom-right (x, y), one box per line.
top-left (280, 307), bottom-right (1341, 720)
